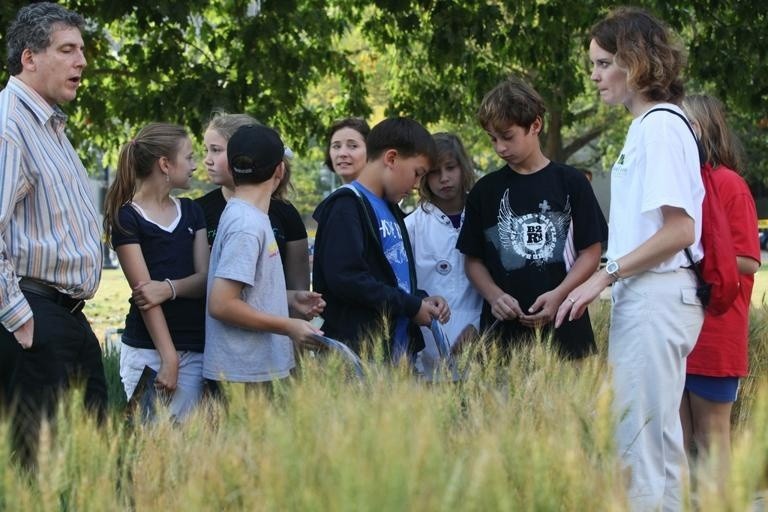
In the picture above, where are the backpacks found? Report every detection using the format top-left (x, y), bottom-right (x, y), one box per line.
top-left (638, 108), bottom-right (741, 318)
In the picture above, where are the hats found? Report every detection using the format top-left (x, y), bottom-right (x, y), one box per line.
top-left (226, 124), bottom-right (287, 175)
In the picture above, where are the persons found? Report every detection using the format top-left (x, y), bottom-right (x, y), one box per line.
top-left (0, 4), bottom-right (109, 475)
top-left (681, 93), bottom-right (762, 499)
top-left (100, 79), bottom-right (607, 427)
top-left (554, 8), bottom-right (706, 512)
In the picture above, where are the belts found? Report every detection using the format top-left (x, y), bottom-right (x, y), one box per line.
top-left (17, 276), bottom-right (86, 316)
